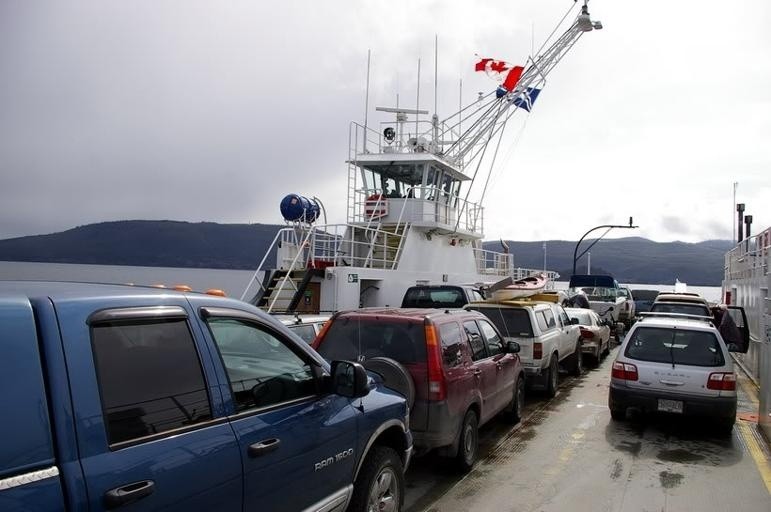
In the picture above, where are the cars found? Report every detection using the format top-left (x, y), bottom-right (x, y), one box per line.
top-left (608, 290), bottom-right (751, 444)
top-left (313, 307), bottom-right (530, 473)
top-left (394, 272), bottom-right (661, 400)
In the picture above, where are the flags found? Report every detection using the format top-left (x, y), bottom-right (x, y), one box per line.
top-left (497, 83), bottom-right (542, 112)
top-left (475, 57), bottom-right (524, 91)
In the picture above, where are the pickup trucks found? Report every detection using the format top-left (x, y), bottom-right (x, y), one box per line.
top-left (0, 275), bottom-right (414, 512)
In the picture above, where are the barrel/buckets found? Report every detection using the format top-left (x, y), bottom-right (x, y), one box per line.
top-left (280, 193), bottom-right (321, 224)
